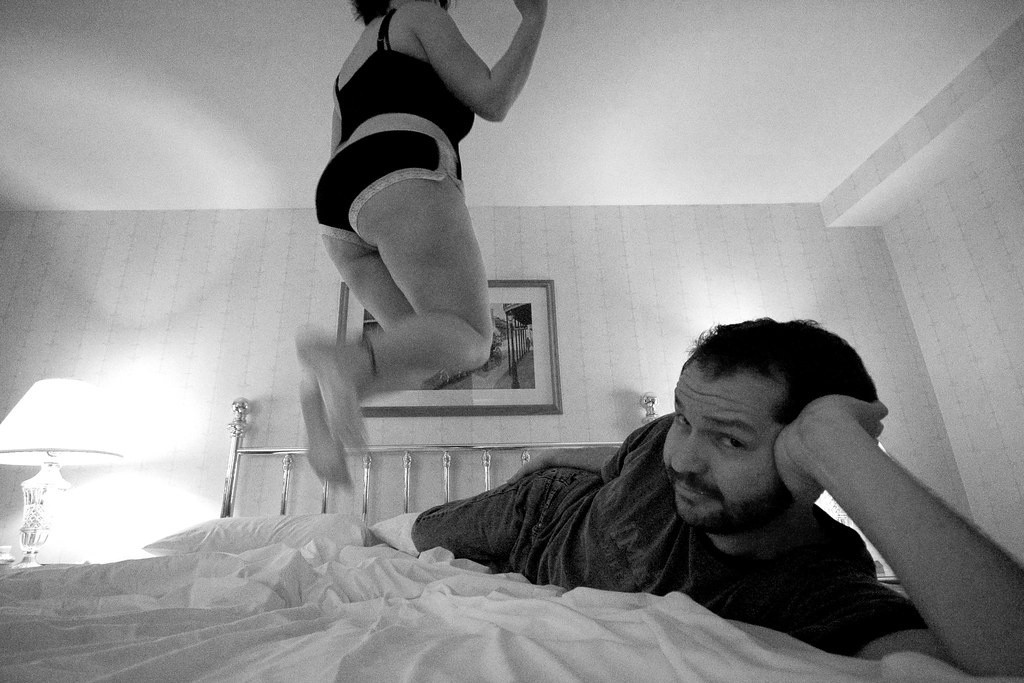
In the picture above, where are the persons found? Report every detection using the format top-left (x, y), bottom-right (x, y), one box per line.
top-left (295, 1), bottom-right (547, 497)
top-left (409, 312), bottom-right (1024, 678)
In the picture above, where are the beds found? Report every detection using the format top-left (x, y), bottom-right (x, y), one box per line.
top-left (0, 394), bottom-right (1024, 683)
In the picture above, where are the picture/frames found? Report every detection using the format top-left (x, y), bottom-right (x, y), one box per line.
top-left (337, 280), bottom-right (564, 418)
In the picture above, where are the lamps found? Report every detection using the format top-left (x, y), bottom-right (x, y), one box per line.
top-left (0, 377), bottom-right (131, 573)
